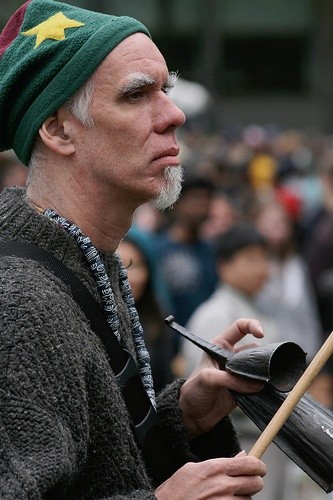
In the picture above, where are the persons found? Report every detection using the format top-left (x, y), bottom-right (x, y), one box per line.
top-left (0, 0), bottom-right (269, 500)
top-left (0, 79), bottom-right (333, 500)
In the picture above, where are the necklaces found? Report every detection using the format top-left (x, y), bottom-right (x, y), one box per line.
top-left (43, 208), bottom-right (156, 412)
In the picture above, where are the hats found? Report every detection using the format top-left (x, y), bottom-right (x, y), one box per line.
top-left (0, 0), bottom-right (154, 167)
